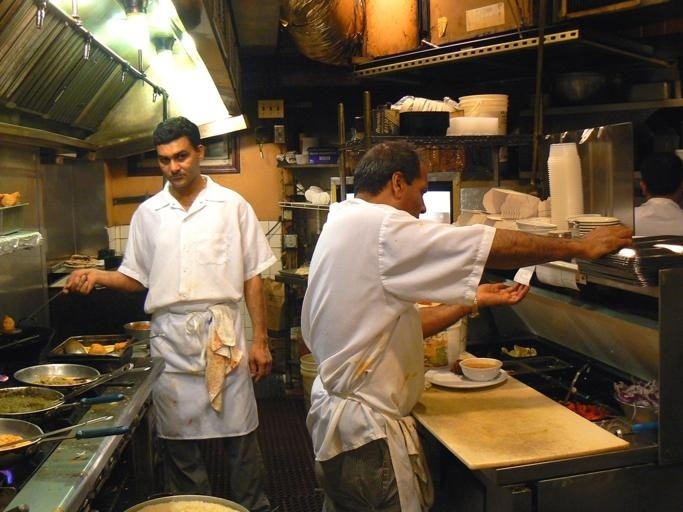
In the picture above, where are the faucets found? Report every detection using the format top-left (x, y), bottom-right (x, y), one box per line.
top-left (562, 361), bottom-right (593, 405)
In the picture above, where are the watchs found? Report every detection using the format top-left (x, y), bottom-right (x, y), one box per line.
top-left (469, 291), bottom-right (480, 320)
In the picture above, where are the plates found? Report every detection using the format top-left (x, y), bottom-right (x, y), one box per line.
top-left (569, 213), bottom-right (620, 237)
top-left (425, 366), bottom-right (507, 389)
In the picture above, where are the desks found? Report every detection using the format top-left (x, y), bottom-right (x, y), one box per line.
top-left (275, 275), bottom-right (307, 390)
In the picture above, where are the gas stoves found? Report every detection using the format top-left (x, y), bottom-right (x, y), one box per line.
top-left (0, 359), bottom-right (168, 512)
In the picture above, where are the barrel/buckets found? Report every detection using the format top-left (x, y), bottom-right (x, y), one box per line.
top-left (300, 354), bottom-right (320, 419)
top-left (414, 300), bottom-right (462, 369)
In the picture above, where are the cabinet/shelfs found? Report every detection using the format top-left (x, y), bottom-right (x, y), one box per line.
top-left (278, 28), bottom-right (683, 272)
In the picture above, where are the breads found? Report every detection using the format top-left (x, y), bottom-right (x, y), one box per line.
top-left (3, 316), bottom-right (15, 332)
top-left (0, 191), bottom-right (23, 208)
top-left (89, 341), bottom-right (107, 356)
top-left (0, 433), bottom-right (32, 451)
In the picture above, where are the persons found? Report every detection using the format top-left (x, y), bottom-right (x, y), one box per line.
top-left (61, 116), bottom-right (280, 512)
top-left (301, 142), bottom-right (634, 512)
top-left (634, 146), bottom-right (683, 237)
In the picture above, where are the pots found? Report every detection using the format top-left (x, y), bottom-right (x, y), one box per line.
top-left (13, 363), bottom-right (153, 387)
top-left (0, 419), bottom-right (128, 452)
top-left (124, 492), bottom-right (249, 512)
top-left (0, 388), bottom-right (123, 417)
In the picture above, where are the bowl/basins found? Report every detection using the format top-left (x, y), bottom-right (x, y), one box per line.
top-left (124, 320), bottom-right (150, 333)
top-left (459, 357), bottom-right (503, 381)
top-left (516, 220), bottom-right (557, 239)
top-left (614, 385), bottom-right (659, 421)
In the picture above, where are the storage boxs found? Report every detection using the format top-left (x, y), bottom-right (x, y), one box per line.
top-left (429, 0), bottom-right (540, 47)
top-left (366, 0), bottom-right (421, 61)
